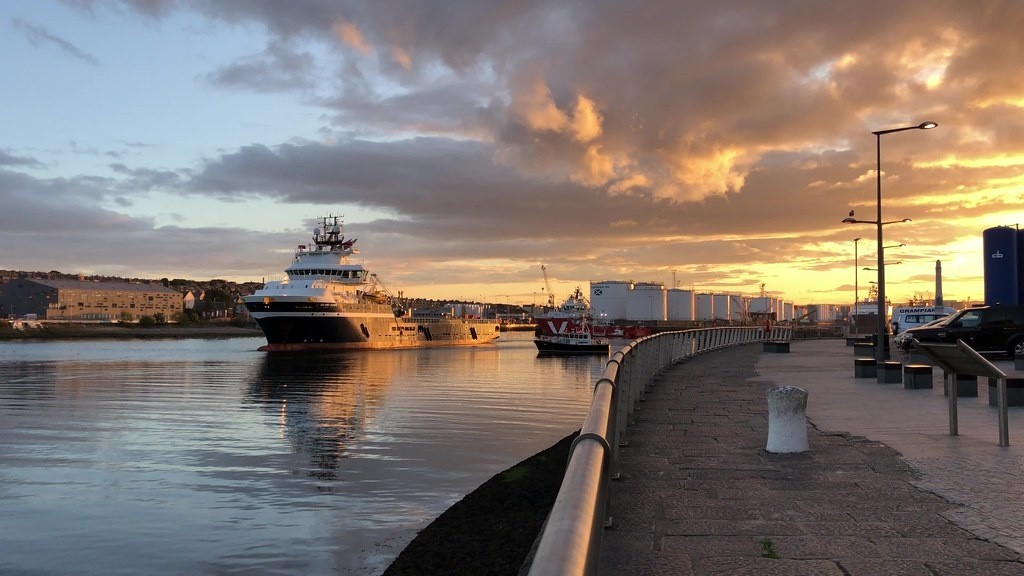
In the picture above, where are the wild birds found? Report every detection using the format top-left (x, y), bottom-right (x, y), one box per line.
top-left (848, 208), bottom-right (855, 219)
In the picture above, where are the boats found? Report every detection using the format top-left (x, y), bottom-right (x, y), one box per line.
top-left (238, 213), bottom-right (502, 352)
top-left (533, 286), bottom-right (609, 358)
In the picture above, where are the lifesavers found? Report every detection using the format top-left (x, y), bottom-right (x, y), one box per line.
top-left (597, 340), bottom-right (601, 344)
top-left (387, 298), bottom-right (391, 305)
top-left (564, 329), bottom-right (568, 333)
top-left (577, 328), bottom-right (580, 331)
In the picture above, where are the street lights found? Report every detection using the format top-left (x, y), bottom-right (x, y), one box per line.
top-left (853, 237), bottom-right (861, 334)
top-left (841, 120), bottom-right (938, 362)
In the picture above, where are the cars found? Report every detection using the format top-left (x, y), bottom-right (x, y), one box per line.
top-left (890, 303), bottom-right (1024, 358)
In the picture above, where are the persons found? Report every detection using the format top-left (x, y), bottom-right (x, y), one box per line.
top-left (765, 319), bottom-right (771, 341)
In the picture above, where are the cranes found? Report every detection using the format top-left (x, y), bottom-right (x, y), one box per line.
top-left (540, 265), bottom-right (554, 308)
